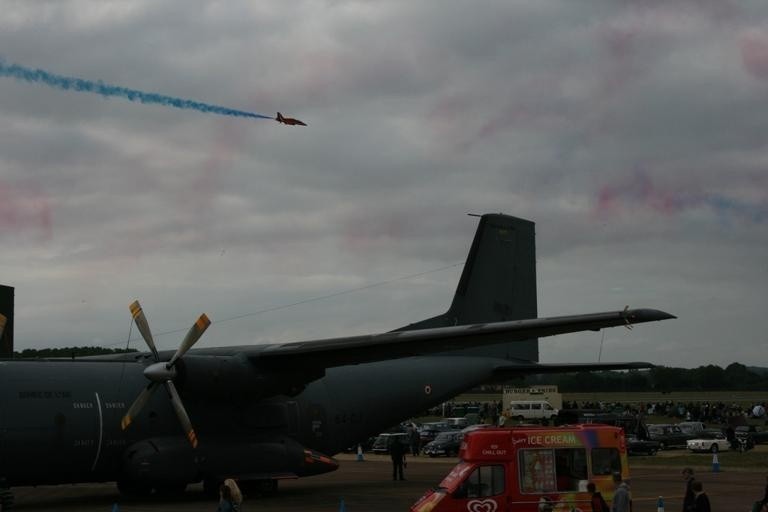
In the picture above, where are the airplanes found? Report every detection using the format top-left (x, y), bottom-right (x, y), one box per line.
top-left (274, 112), bottom-right (307, 128)
top-left (0, 211), bottom-right (680, 512)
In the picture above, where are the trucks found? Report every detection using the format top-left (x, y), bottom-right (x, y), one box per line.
top-left (411, 424), bottom-right (631, 512)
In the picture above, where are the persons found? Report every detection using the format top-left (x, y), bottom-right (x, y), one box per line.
top-left (408, 427), bottom-right (421, 457)
top-left (687, 478), bottom-right (711, 512)
top-left (752, 487), bottom-right (768, 512)
top-left (429, 398), bottom-right (504, 424)
top-left (216, 486), bottom-right (238, 512)
top-left (678, 466), bottom-right (695, 510)
top-left (389, 436), bottom-right (409, 481)
top-left (584, 483), bottom-right (609, 512)
top-left (220, 479), bottom-right (242, 509)
top-left (609, 471), bottom-right (631, 511)
top-left (564, 399), bottom-right (767, 426)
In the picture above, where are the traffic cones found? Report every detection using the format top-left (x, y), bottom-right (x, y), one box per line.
top-left (655, 496), bottom-right (666, 512)
top-left (712, 455), bottom-right (721, 472)
top-left (338, 497), bottom-right (346, 512)
top-left (112, 503), bottom-right (119, 512)
top-left (357, 445), bottom-right (364, 461)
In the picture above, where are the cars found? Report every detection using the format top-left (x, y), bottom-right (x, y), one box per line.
top-left (372, 416), bottom-right (497, 458)
top-left (621, 422), bottom-right (756, 454)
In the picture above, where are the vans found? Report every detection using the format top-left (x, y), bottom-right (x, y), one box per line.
top-left (510, 401), bottom-right (559, 420)
